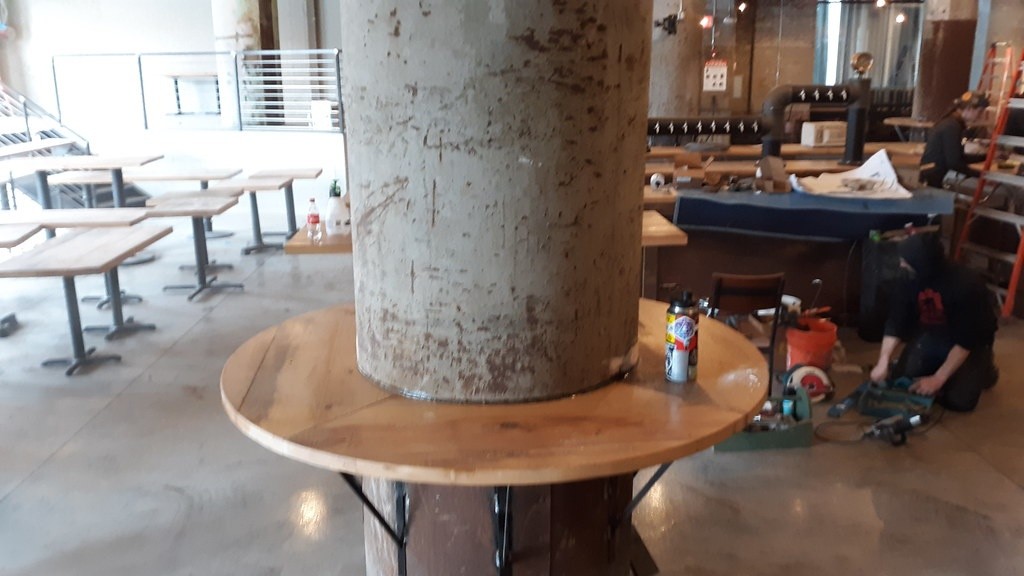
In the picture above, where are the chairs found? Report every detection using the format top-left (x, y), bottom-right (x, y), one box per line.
top-left (707, 271), bottom-right (786, 397)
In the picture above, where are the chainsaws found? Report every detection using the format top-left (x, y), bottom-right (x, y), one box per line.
top-left (783, 362), bottom-right (837, 406)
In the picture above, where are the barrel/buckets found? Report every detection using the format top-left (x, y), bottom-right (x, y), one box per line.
top-left (786, 318), bottom-right (837, 373)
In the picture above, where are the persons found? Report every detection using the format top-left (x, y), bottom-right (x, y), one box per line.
top-left (919, 91), bottom-right (989, 188)
top-left (871, 232), bottom-right (998, 412)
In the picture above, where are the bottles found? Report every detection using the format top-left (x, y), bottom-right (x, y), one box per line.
top-left (650, 172), bottom-right (665, 189)
top-left (326, 179), bottom-right (350, 237)
top-left (666, 291), bottom-right (699, 383)
top-left (306, 197), bottom-right (322, 242)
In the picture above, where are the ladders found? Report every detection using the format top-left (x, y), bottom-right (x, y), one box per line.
top-left (975, 41), bottom-right (1015, 148)
top-left (956, 48), bottom-right (1024, 325)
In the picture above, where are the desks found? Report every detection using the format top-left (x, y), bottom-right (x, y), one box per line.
top-left (0, 152), bottom-right (324, 380)
top-left (286, 192), bottom-right (353, 255)
top-left (641, 116), bottom-right (1024, 302)
top-left (161, 69), bottom-right (222, 117)
top-left (218, 294), bottom-right (770, 576)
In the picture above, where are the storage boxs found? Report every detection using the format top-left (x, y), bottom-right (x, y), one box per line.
top-left (713, 420), bottom-right (812, 453)
top-left (802, 120), bottom-right (848, 148)
